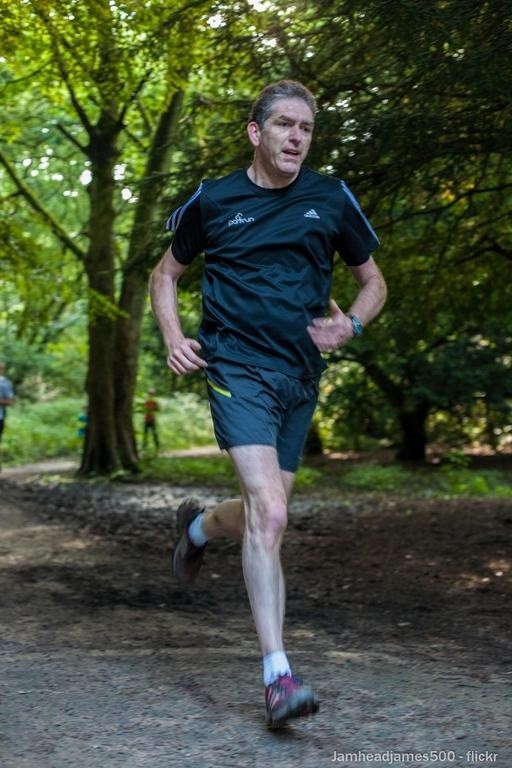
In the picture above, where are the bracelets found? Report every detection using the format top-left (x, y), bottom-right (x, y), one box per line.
top-left (347, 312), bottom-right (363, 339)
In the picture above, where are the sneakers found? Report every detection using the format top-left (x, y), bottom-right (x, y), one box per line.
top-left (263, 674), bottom-right (322, 727)
top-left (170, 496), bottom-right (206, 585)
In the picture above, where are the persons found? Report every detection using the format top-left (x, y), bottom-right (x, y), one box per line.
top-left (151, 82), bottom-right (388, 727)
top-left (77, 403), bottom-right (88, 457)
top-left (143, 386), bottom-right (159, 451)
top-left (0, 359), bottom-right (16, 442)
